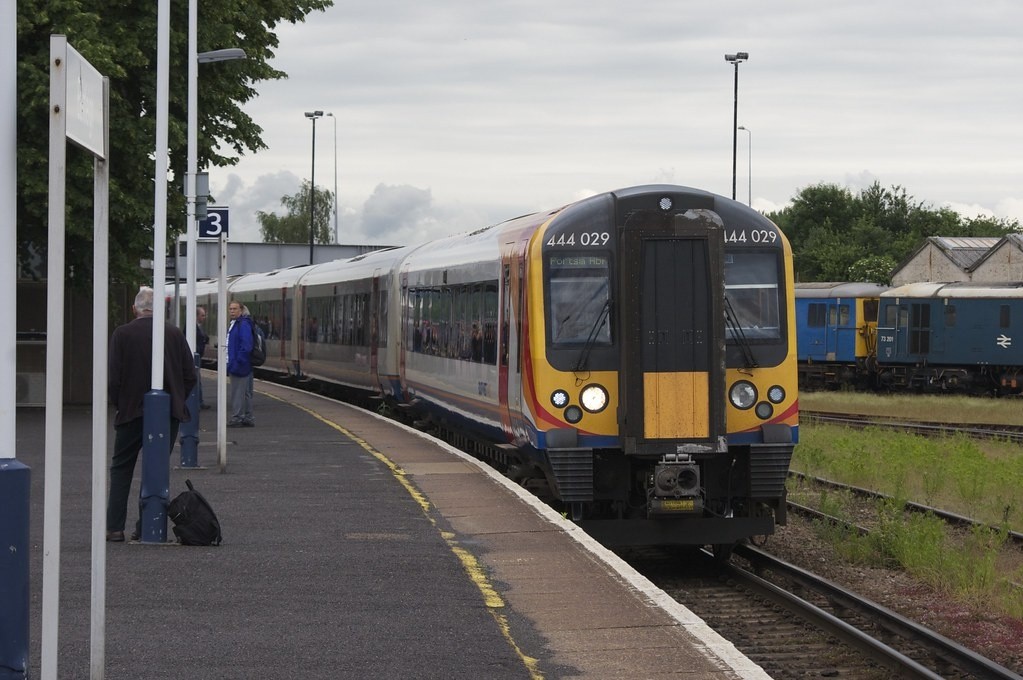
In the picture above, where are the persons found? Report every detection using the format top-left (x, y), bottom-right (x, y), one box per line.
top-left (106, 287), bottom-right (198, 541)
top-left (224, 301), bottom-right (266, 428)
top-left (183, 306), bottom-right (212, 410)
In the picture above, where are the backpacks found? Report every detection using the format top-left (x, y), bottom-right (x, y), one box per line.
top-left (237, 320), bottom-right (266, 366)
top-left (168, 480), bottom-right (222, 545)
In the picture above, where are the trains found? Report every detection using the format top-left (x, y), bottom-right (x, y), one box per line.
top-left (165, 182), bottom-right (804, 564)
top-left (790, 280), bottom-right (1023, 403)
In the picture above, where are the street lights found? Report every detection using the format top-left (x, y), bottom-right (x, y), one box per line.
top-left (183, 47), bottom-right (248, 367)
top-left (327, 110), bottom-right (339, 245)
top-left (304, 109), bottom-right (323, 265)
top-left (737, 126), bottom-right (752, 206)
top-left (724, 50), bottom-right (749, 200)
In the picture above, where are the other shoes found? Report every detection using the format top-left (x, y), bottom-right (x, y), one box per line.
top-left (199, 402), bottom-right (210, 409)
top-left (107, 531), bottom-right (124, 541)
top-left (131, 527), bottom-right (142, 540)
top-left (227, 418), bottom-right (243, 426)
top-left (242, 418), bottom-right (255, 426)
